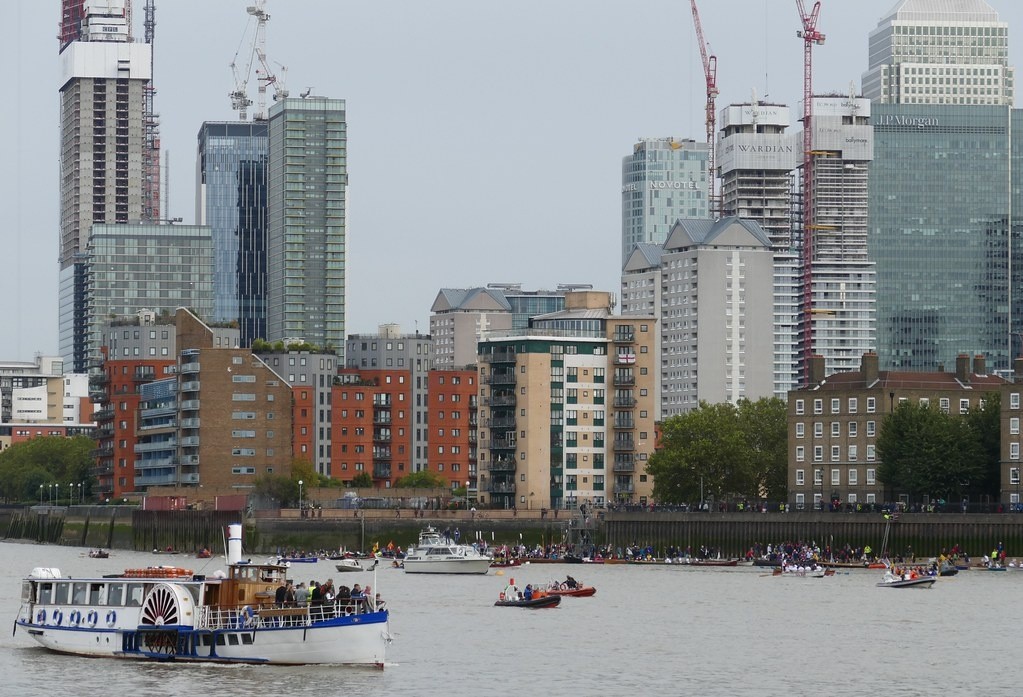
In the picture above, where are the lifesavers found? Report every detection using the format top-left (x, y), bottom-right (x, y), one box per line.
top-left (71, 609), bottom-right (80, 627)
top-left (240, 606), bottom-right (253, 626)
top-left (106, 609), bottom-right (116, 628)
top-left (37, 608), bottom-right (45, 626)
top-left (53, 608), bottom-right (62, 626)
top-left (560, 584), bottom-right (567, 591)
top-left (87, 609), bottom-right (97, 628)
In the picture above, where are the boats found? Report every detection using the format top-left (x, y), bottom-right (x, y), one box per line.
top-left (875, 573), bottom-right (937, 588)
top-left (195, 554), bottom-right (211, 559)
top-left (309, 555), bottom-right (325, 558)
top-left (373, 551), bottom-right (404, 561)
top-left (277, 553), bottom-right (320, 562)
top-left (404, 523), bottom-right (495, 573)
top-left (773, 568), bottom-right (835, 578)
top-left (925, 558), bottom-right (959, 576)
top-left (336, 559), bottom-right (363, 573)
top-left (87, 551), bottom-right (109, 559)
top-left (865, 561), bottom-right (887, 569)
top-left (988, 565), bottom-right (1007, 572)
top-left (547, 585), bottom-right (596, 597)
top-left (328, 552), bottom-right (350, 561)
top-left (491, 557), bottom-right (524, 569)
top-left (494, 592), bottom-right (562, 609)
top-left (956, 566), bottom-right (968, 570)
top-left (562, 555), bottom-right (738, 567)
top-left (152, 551), bottom-right (181, 554)
top-left (14, 524), bottom-right (392, 669)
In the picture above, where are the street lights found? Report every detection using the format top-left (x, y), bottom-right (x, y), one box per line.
top-left (49, 485), bottom-right (52, 505)
top-left (820, 468), bottom-right (824, 512)
top-left (55, 483), bottom-right (58, 506)
top-left (78, 484), bottom-right (81, 505)
top-left (69, 483), bottom-right (73, 506)
top-left (299, 480), bottom-right (302, 519)
top-left (40, 485), bottom-right (43, 506)
top-left (466, 480), bottom-right (470, 509)
top-left (571, 479), bottom-right (573, 516)
top-left (699, 474), bottom-right (704, 509)
top-left (1015, 468), bottom-right (1019, 514)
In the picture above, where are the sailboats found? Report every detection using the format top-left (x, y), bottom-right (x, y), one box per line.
top-left (349, 513), bottom-right (374, 559)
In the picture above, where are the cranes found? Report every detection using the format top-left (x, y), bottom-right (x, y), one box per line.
top-left (229, 0), bottom-right (290, 121)
top-left (795, 0), bottom-right (827, 384)
top-left (691, 0), bottom-right (728, 218)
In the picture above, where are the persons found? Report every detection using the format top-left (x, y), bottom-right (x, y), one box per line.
top-left (525, 584), bottom-right (534, 600)
top-left (443, 527), bottom-right (451, 546)
top-left (472, 539), bottom-right (1007, 581)
top-left (280, 545), bottom-right (405, 559)
top-left (298, 494), bottom-right (1023, 519)
top-left (276, 578), bottom-right (384, 627)
top-left (563, 575), bottom-right (580, 590)
top-left (532, 586), bottom-right (548, 599)
top-left (454, 527), bottom-right (460, 546)
